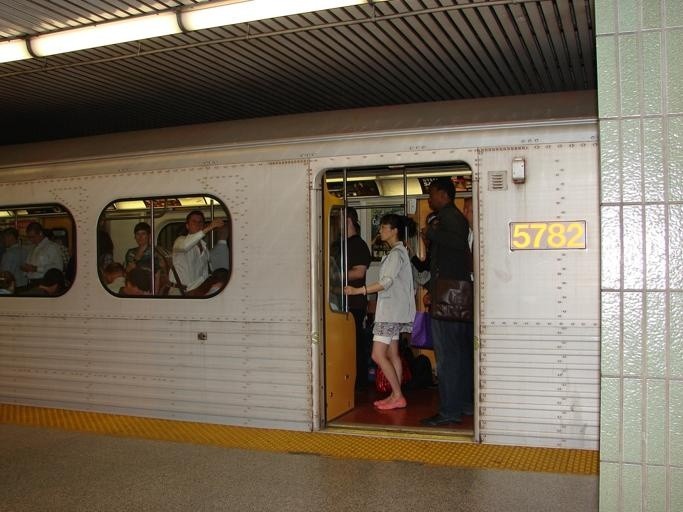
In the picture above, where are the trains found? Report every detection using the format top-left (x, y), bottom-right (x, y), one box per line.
top-left (3, 115), bottom-right (602, 455)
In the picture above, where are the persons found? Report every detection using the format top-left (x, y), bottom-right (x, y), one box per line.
top-left (0, 207), bottom-right (230, 298)
top-left (331, 173), bottom-right (473, 426)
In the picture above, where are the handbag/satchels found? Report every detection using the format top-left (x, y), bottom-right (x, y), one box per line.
top-left (375, 357), bottom-right (413, 394)
top-left (430, 277), bottom-right (473, 323)
top-left (409, 311), bottom-right (433, 350)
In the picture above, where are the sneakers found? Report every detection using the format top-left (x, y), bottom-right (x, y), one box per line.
top-left (374, 397), bottom-right (407, 410)
